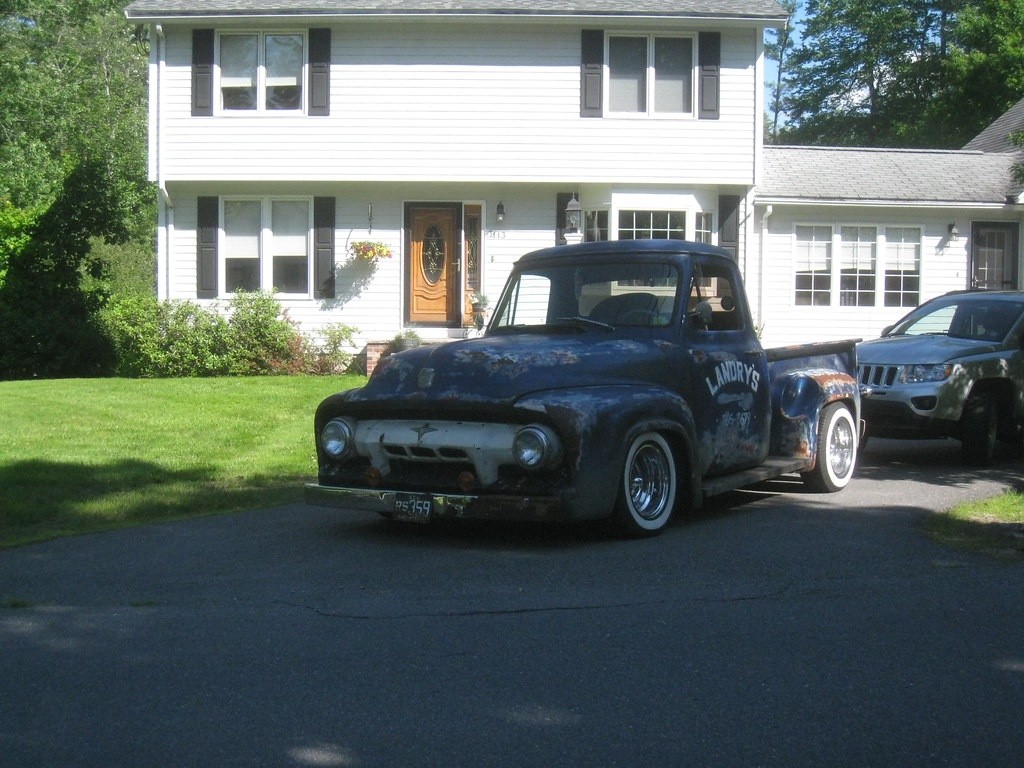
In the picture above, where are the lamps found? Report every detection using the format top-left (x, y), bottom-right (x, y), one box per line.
top-left (496, 204), bottom-right (506, 222)
top-left (948, 222), bottom-right (959, 240)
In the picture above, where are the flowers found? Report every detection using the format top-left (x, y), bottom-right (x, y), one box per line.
top-left (350, 241), bottom-right (393, 259)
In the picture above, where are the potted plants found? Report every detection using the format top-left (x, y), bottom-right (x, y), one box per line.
top-left (398, 321), bottom-right (419, 352)
top-left (472, 291), bottom-right (488, 312)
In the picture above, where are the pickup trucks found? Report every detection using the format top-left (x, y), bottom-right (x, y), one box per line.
top-left (300, 238), bottom-right (870, 537)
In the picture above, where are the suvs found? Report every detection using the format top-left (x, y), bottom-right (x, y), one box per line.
top-left (856, 286), bottom-right (1024, 466)
